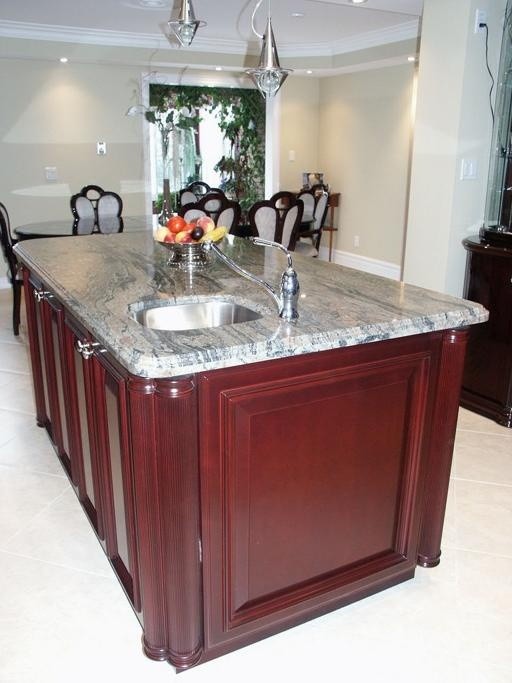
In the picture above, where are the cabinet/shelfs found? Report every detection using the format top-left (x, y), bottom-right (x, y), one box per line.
top-left (26, 271), bottom-right (62, 457)
top-left (459, 236), bottom-right (512, 432)
top-left (63, 312), bottom-right (141, 616)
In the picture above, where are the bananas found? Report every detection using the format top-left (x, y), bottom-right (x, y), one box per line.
top-left (198, 226), bottom-right (227, 243)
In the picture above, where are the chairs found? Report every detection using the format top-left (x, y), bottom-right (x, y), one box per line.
top-left (0, 203), bottom-right (25, 336)
top-left (71, 184), bottom-right (120, 216)
top-left (176, 181), bottom-right (223, 204)
top-left (72, 217), bottom-right (123, 233)
top-left (249, 192), bottom-right (303, 249)
top-left (181, 192), bottom-right (242, 236)
top-left (295, 184), bottom-right (331, 258)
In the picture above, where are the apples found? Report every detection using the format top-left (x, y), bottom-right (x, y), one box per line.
top-left (183, 223), bottom-right (196, 232)
top-left (167, 215), bottom-right (186, 233)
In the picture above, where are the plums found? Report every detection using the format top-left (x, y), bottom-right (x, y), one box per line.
top-left (191, 227), bottom-right (204, 240)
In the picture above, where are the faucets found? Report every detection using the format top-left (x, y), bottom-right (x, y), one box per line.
top-left (203, 236), bottom-right (301, 320)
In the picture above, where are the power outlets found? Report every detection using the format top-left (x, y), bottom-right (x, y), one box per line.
top-left (475, 10), bottom-right (488, 34)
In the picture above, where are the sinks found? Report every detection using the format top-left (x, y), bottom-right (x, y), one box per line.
top-left (136, 301), bottom-right (264, 331)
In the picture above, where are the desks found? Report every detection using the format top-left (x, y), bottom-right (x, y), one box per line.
top-left (15, 214), bottom-right (316, 239)
top-left (285, 188), bottom-right (339, 261)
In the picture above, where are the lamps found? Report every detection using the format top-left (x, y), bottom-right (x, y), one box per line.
top-left (167, 0), bottom-right (207, 47)
top-left (245, 0), bottom-right (294, 99)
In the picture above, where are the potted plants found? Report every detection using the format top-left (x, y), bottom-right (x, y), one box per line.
top-left (124, 87), bottom-right (206, 224)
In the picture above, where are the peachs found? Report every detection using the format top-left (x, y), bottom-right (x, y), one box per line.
top-left (164, 232), bottom-right (176, 244)
top-left (175, 231), bottom-right (192, 244)
top-left (154, 226), bottom-right (172, 243)
top-left (196, 216), bottom-right (215, 234)
